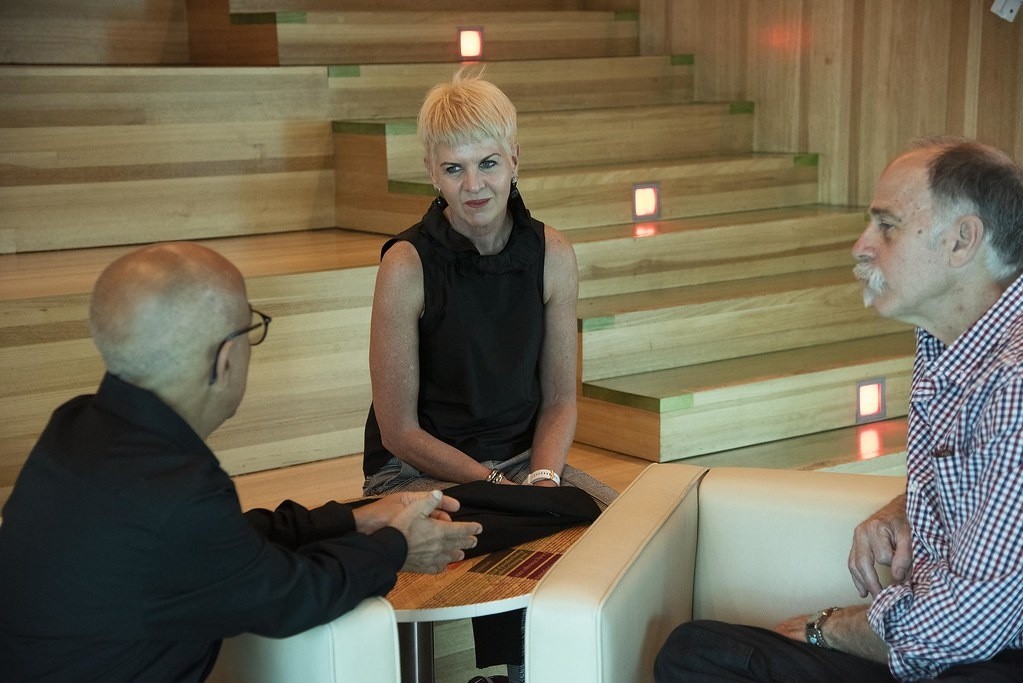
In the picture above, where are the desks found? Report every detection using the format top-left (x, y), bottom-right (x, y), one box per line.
top-left (331, 494), bottom-right (589, 683)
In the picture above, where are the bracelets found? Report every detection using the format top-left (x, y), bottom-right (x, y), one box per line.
top-left (523, 469), bottom-right (560, 486)
top-left (485, 469), bottom-right (504, 484)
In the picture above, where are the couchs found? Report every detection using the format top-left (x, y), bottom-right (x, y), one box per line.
top-left (526, 461), bottom-right (952, 683)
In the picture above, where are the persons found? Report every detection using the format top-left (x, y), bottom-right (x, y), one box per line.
top-left (0, 240), bottom-right (482, 683)
top-left (364, 67), bottom-right (619, 663)
top-left (652, 135), bottom-right (1023, 683)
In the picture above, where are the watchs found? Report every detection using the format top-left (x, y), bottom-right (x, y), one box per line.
top-left (808, 607), bottom-right (838, 646)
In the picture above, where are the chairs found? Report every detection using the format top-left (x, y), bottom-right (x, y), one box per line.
top-left (198, 595), bottom-right (402, 683)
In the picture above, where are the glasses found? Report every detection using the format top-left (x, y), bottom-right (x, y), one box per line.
top-left (208, 309), bottom-right (272, 385)
